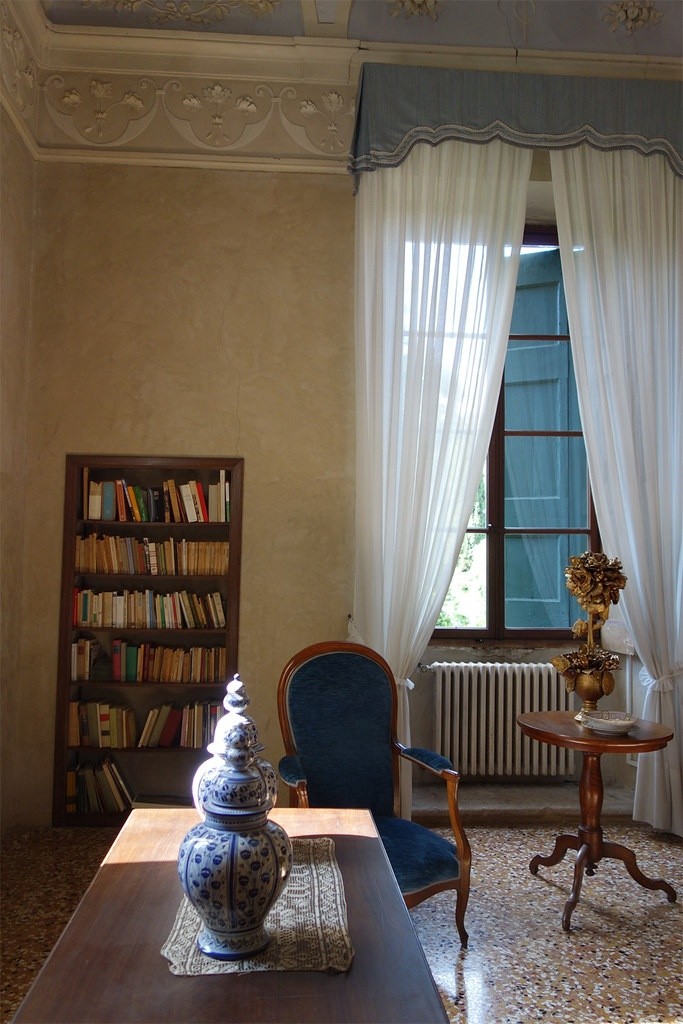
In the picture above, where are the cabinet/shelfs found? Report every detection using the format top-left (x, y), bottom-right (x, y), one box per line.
top-left (48, 452), bottom-right (245, 830)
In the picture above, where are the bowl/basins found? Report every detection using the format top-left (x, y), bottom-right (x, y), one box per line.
top-left (582, 710), bottom-right (639, 730)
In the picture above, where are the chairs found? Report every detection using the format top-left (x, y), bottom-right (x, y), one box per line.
top-left (276, 641), bottom-right (473, 952)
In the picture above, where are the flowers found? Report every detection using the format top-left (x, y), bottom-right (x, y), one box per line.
top-left (549, 549), bottom-right (629, 671)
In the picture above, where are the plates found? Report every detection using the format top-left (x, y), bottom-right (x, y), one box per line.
top-left (580, 720), bottom-right (641, 737)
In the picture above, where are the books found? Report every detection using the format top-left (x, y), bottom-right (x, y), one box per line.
top-left (75, 532), bottom-right (229, 575)
top-left (82, 466), bottom-right (230, 521)
top-left (73, 586), bottom-right (225, 628)
top-left (70, 638), bottom-right (225, 682)
top-left (65, 756), bottom-right (193, 813)
top-left (68, 698), bottom-right (223, 747)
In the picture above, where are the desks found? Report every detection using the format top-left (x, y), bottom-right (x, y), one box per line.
top-left (515, 712), bottom-right (679, 934)
top-left (12, 810), bottom-right (453, 1024)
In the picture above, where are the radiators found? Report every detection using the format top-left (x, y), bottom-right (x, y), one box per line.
top-left (431, 661), bottom-right (575, 778)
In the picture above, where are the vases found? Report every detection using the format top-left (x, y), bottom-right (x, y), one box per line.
top-left (566, 671), bottom-right (615, 722)
